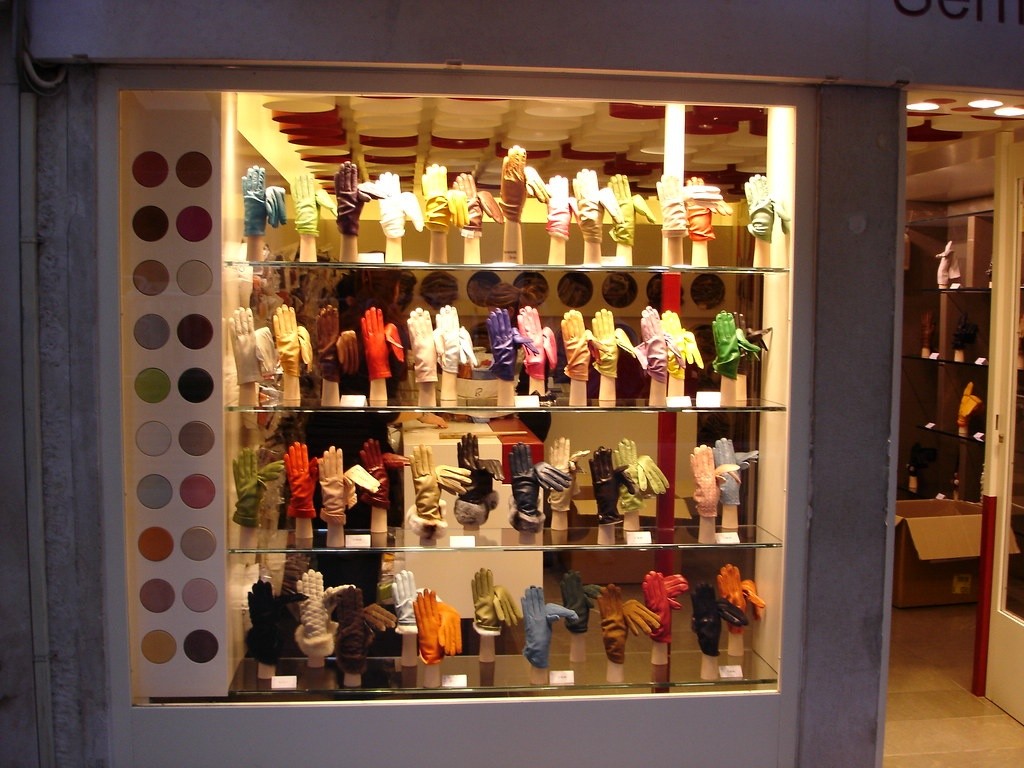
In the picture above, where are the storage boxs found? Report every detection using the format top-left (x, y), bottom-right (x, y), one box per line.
top-left (893, 499), bottom-right (1021, 608)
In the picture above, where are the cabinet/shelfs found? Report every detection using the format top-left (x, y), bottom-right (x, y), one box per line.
top-left (102, 64), bottom-right (815, 695)
top-left (893, 190), bottom-right (1024, 685)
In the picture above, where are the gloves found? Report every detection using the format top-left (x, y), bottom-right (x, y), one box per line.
top-left (229, 143), bottom-right (798, 271)
top-left (906, 238), bottom-right (994, 502)
top-left (227, 425), bottom-right (762, 525)
top-left (241, 555), bottom-right (764, 670)
top-left (224, 292), bottom-right (794, 413)
